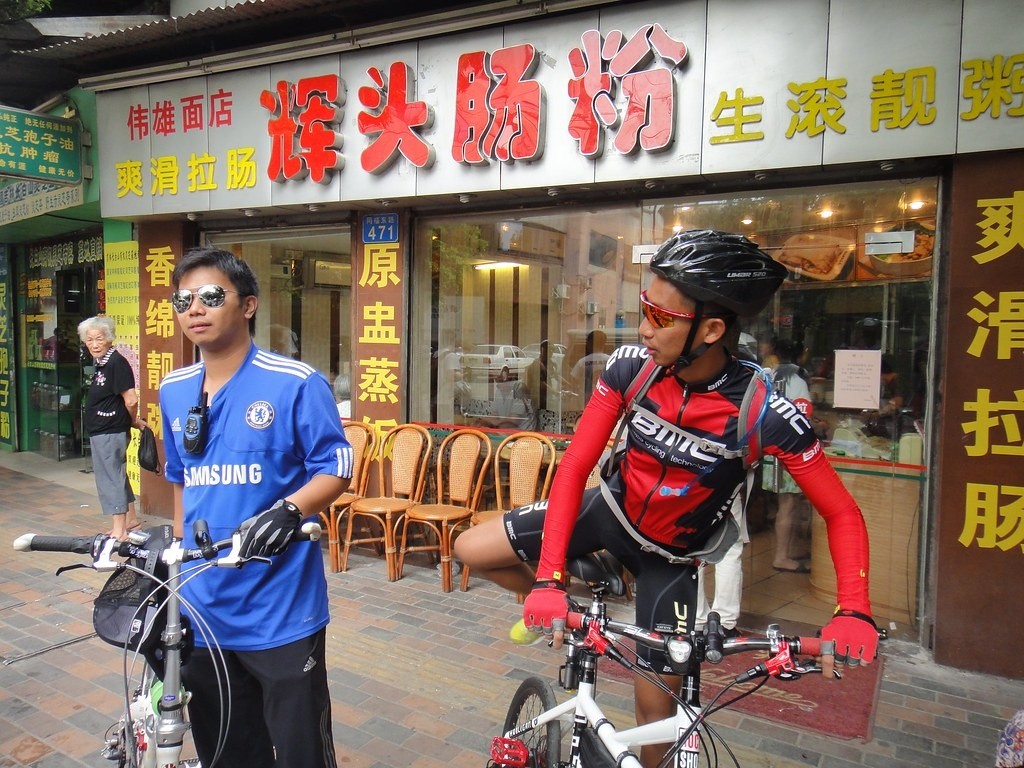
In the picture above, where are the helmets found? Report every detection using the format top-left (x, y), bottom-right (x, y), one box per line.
top-left (648, 227), bottom-right (789, 316)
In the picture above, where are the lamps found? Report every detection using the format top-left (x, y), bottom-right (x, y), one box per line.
top-left (471, 261), bottom-right (521, 271)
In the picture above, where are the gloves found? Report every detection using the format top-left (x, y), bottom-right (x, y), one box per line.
top-left (234, 498), bottom-right (304, 560)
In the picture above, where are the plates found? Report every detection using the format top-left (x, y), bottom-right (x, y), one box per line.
top-left (771, 233), bottom-right (853, 280)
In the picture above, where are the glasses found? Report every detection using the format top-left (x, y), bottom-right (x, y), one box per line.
top-left (171, 284), bottom-right (247, 314)
top-left (639, 289), bottom-right (728, 328)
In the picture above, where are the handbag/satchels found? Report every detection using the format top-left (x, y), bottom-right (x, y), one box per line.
top-left (137, 418), bottom-right (163, 476)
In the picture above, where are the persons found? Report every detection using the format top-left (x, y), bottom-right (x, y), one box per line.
top-left (570, 330), bottom-right (611, 388)
top-left (77, 316), bottom-right (153, 542)
top-left (527, 340), bottom-right (571, 396)
top-left (849, 354), bottom-right (923, 443)
top-left (454, 229), bottom-right (879, 768)
top-left (760, 339), bottom-right (811, 573)
top-left (694, 488), bottom-right (744, 638)
top-left (758, 329), bottom-right (780, 369)
top-left (159, 249), bottom-right (354, 768)
top-left (444, 372), bottom-right (472, 404)
top-left (443, 348), bottom-right (460, 368)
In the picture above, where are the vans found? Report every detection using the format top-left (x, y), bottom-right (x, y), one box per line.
top-left (569, 328), bottom-right (758, 374)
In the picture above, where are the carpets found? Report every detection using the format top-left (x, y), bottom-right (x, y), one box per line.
top-left (598, 627), bottom-right (884, 744)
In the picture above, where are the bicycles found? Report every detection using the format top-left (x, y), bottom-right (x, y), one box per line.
top-left (12, 522), bottom-right (325, 768)
top-left (484, 550), bottom-right (888, 765)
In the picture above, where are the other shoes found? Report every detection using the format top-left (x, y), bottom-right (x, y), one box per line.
top-left (507, 618), bottom-right (540, 646)
top-left (125, 521), bottom-right (143, 535)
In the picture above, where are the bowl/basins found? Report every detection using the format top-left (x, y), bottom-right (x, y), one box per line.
top-left (869, 222), bottom-right (934, 276)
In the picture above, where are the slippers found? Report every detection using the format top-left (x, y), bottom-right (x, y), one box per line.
top-left (770, 558), bottom-right (810, 573)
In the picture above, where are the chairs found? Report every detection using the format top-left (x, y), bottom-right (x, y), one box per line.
top-left (343, 423), bottom-right (434, 582)
top-left (461, 431), bottom-right (557, 605)
top-left (318, 421), bottom-right (379, 574)
top-left (397, 429), bottom-right (492, 596)
top-left (583, 439), bottom-right (633, 604)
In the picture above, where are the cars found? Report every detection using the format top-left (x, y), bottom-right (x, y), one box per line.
top-left (461, 343), bottom-right (536, 382)
top-left (522, 341), bottom-right (572, 369)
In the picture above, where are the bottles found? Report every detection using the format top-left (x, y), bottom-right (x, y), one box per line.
top-left (32, 429), bottom-right (66, 459)
top-left (149, 679), bottom-right (187, 717)
top-left (31, 382), bottom-right (67, 411)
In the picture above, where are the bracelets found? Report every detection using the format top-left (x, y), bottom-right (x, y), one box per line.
top-left (832, 609), bottom-right (877, 631)
top-left (531, 581), bottom-right (566, 592)
